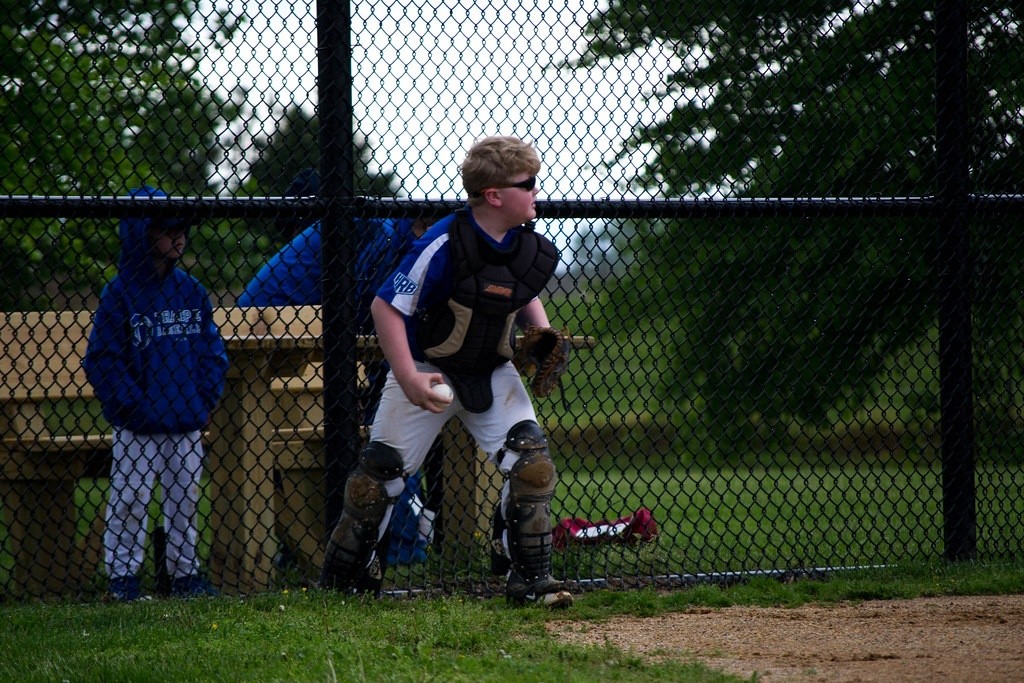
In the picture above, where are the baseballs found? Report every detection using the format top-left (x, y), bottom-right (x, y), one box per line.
top-left (430, 382), bottom-right (455, 410)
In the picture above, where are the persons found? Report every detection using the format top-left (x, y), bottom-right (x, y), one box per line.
top-left (236, 215), bottom-right (439, 426)
top-left (83, 186), bottom-right (230, 605)
top-left (319, 139), bottom-right (574, 609)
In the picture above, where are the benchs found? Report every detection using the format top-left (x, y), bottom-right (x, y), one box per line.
top-left (0, 304), bottom-right (380, 604)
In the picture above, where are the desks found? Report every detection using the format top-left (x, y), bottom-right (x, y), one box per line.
top-left (208, 334), bottom-right (599, 591)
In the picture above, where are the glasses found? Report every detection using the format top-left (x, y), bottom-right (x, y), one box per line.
top-left (479, 178), bottom-right (535, 193)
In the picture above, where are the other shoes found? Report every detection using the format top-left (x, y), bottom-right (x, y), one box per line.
top-left (173, 576), bottom-right (219, 600)
top-left (107, 577), bottom-right (152, 602)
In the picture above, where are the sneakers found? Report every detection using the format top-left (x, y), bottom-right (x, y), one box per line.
top-left (511, 575), bottom-right (573, 609)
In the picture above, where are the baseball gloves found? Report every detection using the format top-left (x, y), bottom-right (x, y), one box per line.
top-left (520, 324), bottom-right (573, 400)
